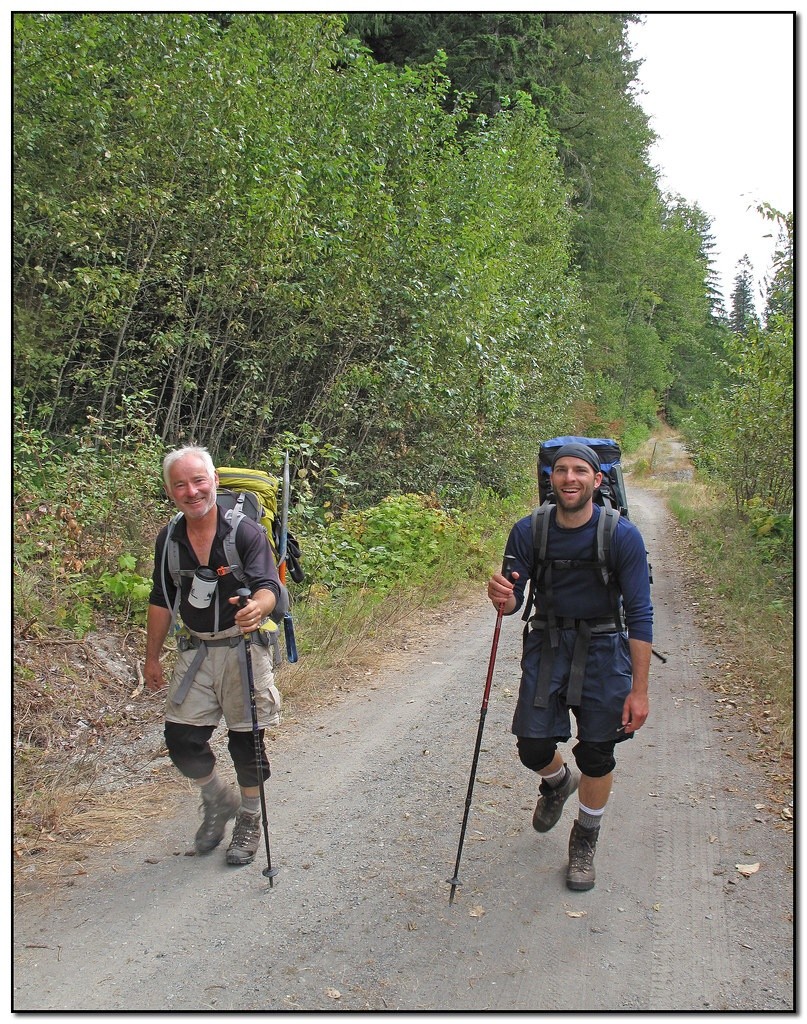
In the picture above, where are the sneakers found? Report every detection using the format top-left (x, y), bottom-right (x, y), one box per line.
top-left (532, 761), bottom-right (581, 834)
top-left (226, 808), bottom-right (261, 865)
top-left (195, 783), bottom-right (243, 852)
top-left (565, 819), bottom-right (601, 891)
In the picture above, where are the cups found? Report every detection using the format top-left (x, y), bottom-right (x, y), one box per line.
top-left (188, 566), bottom-right (218, 609)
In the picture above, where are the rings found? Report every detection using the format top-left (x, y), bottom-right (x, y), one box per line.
top-left (143, 444), bottom-right (290, 866)
top-left (251, 618), bottom-right (255, 624)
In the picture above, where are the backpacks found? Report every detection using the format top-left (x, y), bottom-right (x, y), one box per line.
top-left (171, 465), bottom-right (278, 646)
top-left (521, 434), bottom-right (627, 635)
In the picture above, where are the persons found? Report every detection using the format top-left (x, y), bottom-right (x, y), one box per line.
top-left (487, 441), bottom-right (655, 894)
